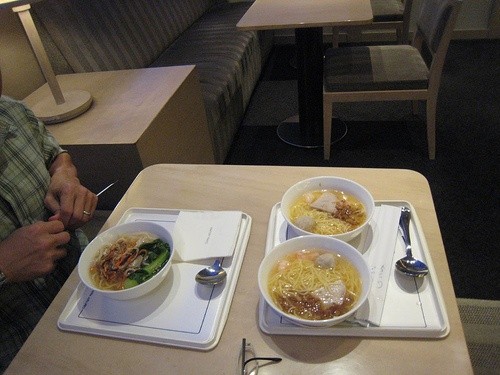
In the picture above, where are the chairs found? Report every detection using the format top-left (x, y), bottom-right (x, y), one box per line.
top-left (323, 0), bottom-right (464, 161)
top-left (456, 297), bottom-right (500, 375)
top-left (331, 0), bottom-right (413, 49)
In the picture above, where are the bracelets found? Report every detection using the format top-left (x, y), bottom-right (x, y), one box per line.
top-left (0, 271), bottom-right (9, 287)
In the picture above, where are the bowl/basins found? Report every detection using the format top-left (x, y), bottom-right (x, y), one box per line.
top-left (280, 176), bottom-right (375, 241)
top-left (257, 235), bottom-right (371, 328)
top-left (77, 221), bottom-right (174, 300)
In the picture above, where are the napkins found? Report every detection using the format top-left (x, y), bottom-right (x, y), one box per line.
top-left (173, 210), bottom-right (243, 262)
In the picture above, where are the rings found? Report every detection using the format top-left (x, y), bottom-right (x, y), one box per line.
top-left (83, 210), bottom-right (91, 215)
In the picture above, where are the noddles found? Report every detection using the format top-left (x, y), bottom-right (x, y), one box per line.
top-left (289, 190), bottom-right (365, 236)
top-left (88, 233), bottom-right (145, 292)
top-left (268, 248), bottom-right (362, 322)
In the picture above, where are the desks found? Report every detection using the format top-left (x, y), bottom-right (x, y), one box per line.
top-left (236, 0), bottom-right (374, 148)
top-left (3, 164), bottom-right (476, 375)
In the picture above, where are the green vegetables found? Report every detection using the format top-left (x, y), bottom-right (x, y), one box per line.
top-left (116, 238), bottom-right (170, 289)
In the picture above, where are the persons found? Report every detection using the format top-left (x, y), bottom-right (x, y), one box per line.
top-left (0, 89), bottom-right (98, 375)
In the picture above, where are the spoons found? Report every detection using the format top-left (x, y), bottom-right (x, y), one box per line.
top-left (195, 257), bottom-right (226, 285)
top-left (394, 207), bottom-right (429, 278)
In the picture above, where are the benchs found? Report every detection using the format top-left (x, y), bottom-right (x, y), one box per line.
top-left (30, 0), bottom-right (275, 164)
